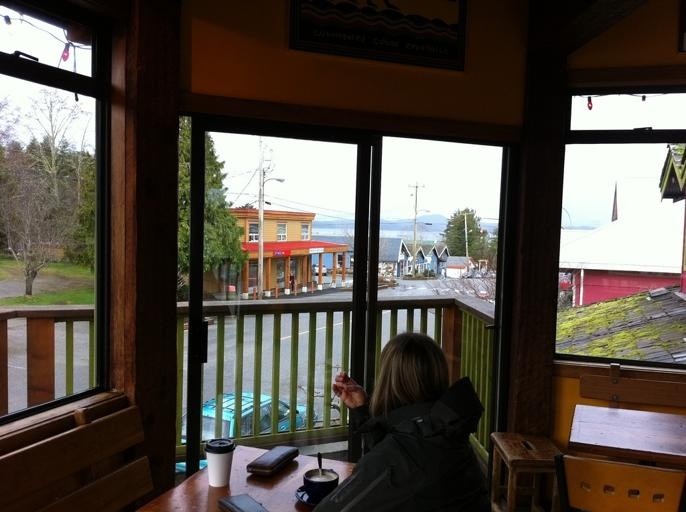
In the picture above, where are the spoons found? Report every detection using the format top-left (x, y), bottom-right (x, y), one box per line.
top-left (316, 451), bottom-right (324, 477)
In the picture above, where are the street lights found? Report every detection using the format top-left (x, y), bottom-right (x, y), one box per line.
top-left (464, 229), bottom-right (477, 273)
top-left (248, 200), bottom-right (272, 206)
top-left (256, 177), bottom-right (286, 299)
top-left (412, 220), bottom-right (432, 279)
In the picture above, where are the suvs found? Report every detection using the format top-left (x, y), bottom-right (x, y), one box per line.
top-left (312, 264), bottom-right (327, 276)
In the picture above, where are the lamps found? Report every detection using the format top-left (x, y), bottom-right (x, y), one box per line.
top-left (587, 96), bottom-right (592, 110)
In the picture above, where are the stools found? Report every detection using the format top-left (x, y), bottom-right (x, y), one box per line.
top-left (489, 431), bottom-right (565, 512)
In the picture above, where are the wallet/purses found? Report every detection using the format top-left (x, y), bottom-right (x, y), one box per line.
top-left (247, 446), bottom-right (299, 477)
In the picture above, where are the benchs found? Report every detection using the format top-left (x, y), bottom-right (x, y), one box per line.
top-left (563, 373), bottom-right (685, 512)
top-left (0, 405), bottom-right (154, 512)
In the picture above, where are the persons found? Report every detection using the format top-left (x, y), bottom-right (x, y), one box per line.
top-left (312, 332), bottom-right (491, 512)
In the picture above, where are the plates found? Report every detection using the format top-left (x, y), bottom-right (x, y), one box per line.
top-left (294, 499), bottom-right (312, 511)
top-left (295, 486), bottom-right (324, 504)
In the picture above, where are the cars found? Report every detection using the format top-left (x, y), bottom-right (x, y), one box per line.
top-left (462, 272), bottom-right (486, 278)
top-left (175, 392), bottom-right (317, 474)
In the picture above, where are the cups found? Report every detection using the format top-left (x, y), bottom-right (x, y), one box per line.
top-left (204, 436), bottom-right (235, 487)
top-left (302, 468), bottom-right (339, 496)
